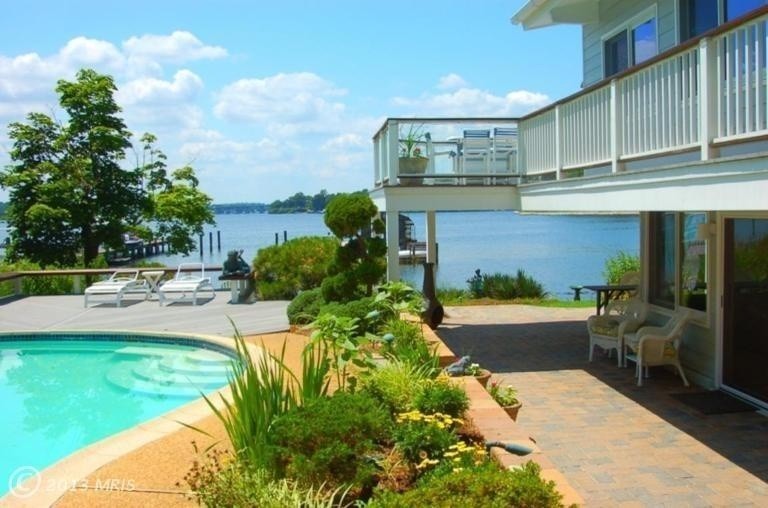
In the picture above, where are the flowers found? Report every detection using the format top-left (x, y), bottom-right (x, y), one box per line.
top-left (488, 377), bottom-right (515, 405)
top-left (470, 360), bottom-right (481, 375)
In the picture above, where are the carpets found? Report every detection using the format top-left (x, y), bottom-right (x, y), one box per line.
top-left (668, 390), bottom-right (758, 415)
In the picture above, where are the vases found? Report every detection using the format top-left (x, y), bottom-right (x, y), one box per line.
top-left (475, 369), bottom-right (492, 388)
top-left (501, 397), bottom-right (521, 422)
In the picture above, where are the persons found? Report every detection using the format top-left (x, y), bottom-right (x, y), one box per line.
top-left (466, 269), bottom-right (482, 285)
top-left (222, 248), bottom-right (251, 277)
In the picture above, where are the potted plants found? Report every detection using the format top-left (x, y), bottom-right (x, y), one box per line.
top-left (398, 122), bottom-right (429, 187)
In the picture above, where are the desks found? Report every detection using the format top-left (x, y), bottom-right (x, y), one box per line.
top-left (582, 285), bottom-right (638, 317)
top-left (142, 270), bottom-right (164, 301)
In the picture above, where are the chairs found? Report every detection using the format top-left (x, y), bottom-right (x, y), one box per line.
top-left (83, 270), bottom-right (153, 308)
top-left (425, 127), bottom-right (518, 185)
top-left (158, 263), bottom-right (216, 305)
top-left (587, 297), bottom-right (647, 368)
top-left (623, 306), bottom-right (692, 389)
